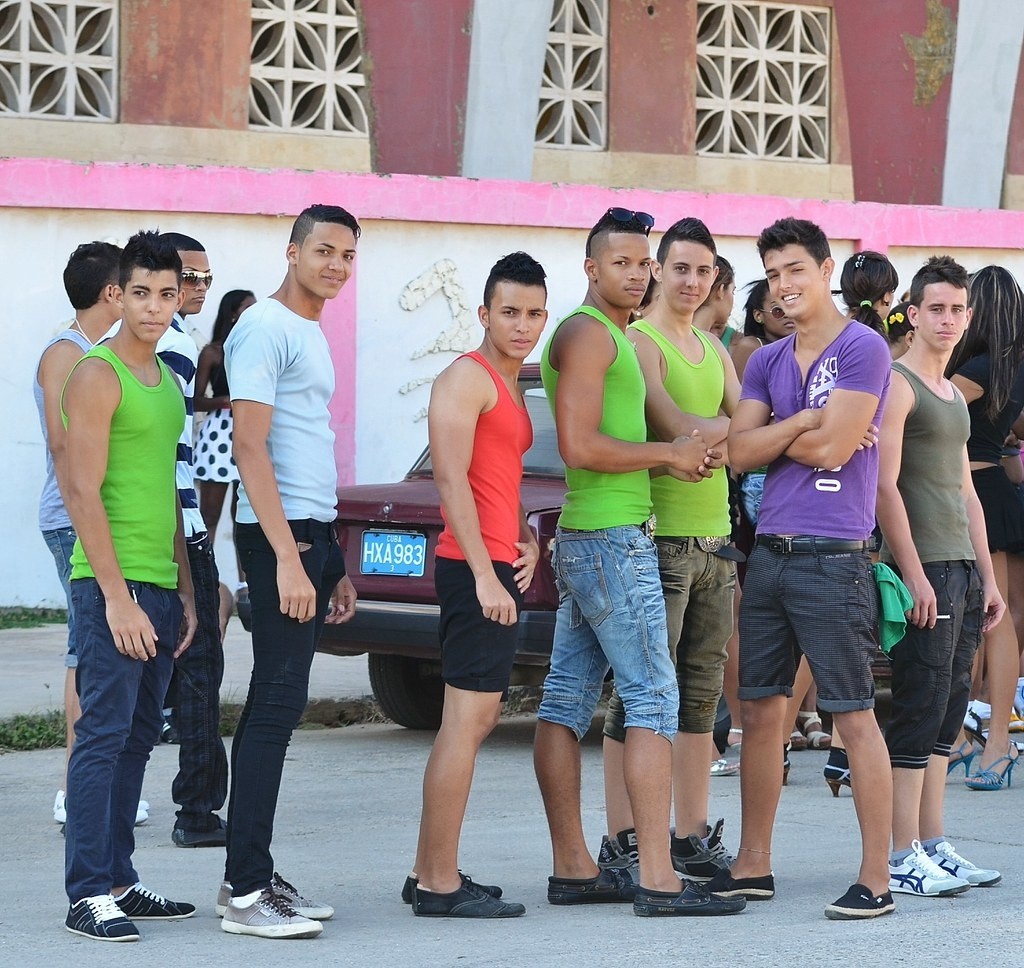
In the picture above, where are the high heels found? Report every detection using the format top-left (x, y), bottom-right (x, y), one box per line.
top-left (947, 740), bottom-right (976, 776)
top-left (823, 765), bottom-right (852, 795)
top-left (964, 708), bottom-right (1023, 755)
top-left (965, 741), bottom-right (1019, 790)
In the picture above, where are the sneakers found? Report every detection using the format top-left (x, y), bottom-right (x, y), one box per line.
top-left (412, 873), bottom-right (527, 919)
top-left (927, 842), bottom-right (1002, 887)
top-left (54, 797), bottom-right (148, 825)
top-left (703, 867), bottom-right (775, 900)
top-left (670, 817), bottom-right (737, 877)
top-left (214, 872), bottom-right (334, 921)
top-left (546, 865), bottom-right (639, 905)
top-left (888, 839), bottom-right (970, 897)
top-left (160, 721), bottom-right (181, 744)
top-left (401, 867), bottom-right (502, 904)
top-left (598, 828), bottom-right (640, 885)
top-left (114, 880), bottom-right (197, 919)
top-left (709, 750), bottom-right (741, 776)
top-left (220, 888), bottom-right (323, 939)
top-left (633, 877), bottom-right (747, 916)
top-left (65, 892), bottom-right (140, 942)
top-left (824, 883), bottom-right (896, 919)
top-left (171, 815), bottom-right (227, 848)
top-left (52, 789), bottom-right (149, 810)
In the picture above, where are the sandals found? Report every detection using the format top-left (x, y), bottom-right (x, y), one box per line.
top-left (799, 710), bottom-right (832, 749)
top-left (789, 729), bottom-right (809, 750)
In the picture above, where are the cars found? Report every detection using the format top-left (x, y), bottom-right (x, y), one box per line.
top-left (237, 362), bottom-right (617, 734)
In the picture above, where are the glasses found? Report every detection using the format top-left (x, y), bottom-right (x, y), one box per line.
top-left (758, 306), bottom-right (785, 320)
top-left (586, 207), bottom-right (654, 257)
top-left (179, 270), bottom-right (212, 290)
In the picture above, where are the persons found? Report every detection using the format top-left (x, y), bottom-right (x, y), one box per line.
top-left (533, 207), bottom-right (1024, 919)
top-left (401, 249), bottom-right (548, 918)
top-left (34, 203), bottom-right (360, 940)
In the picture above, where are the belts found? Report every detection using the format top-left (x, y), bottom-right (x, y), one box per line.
top-left (653, 536), bottom-right (747, 562)
top-left (758, 533), bottom-right (876, 554)
top-left (644, 512), bottom-right (656, 536)
